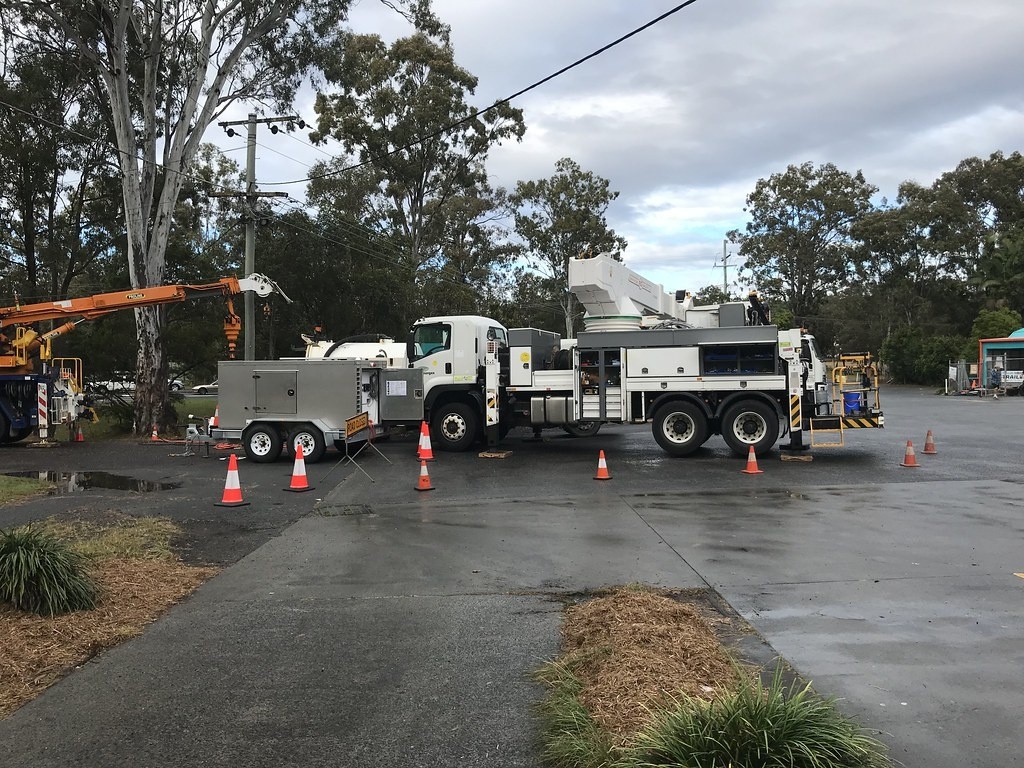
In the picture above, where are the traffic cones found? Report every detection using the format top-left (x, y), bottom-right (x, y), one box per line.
top-left (217, 455), bottom-right (246, 505)
top-left (415, 460), bottom-right (434, 490)
top-left (416, 422), bottom-right (434, 461)
top-left (592, 452), bottom-right (612, 482)
top-left (741, 442), bottom-right (766, 473)
top-left (148, 425), bottom-right (159, 439)
top-left (919, 427), bottom-right (939, 457)
top-left (75, 425), bottom-right (85, 440)
top-left (898, 439), bottom-right (921, 469)
top-left (287, 444), bottom-right (311, 493)
top-left (210, 405), bottom-right (222, 428)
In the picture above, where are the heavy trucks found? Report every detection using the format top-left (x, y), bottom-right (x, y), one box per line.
top-left (214, 314), bottom-right (884, 462)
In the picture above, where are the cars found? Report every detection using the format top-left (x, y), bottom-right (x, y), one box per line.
top-left (90, 375), bottom-right (185, 393)
top-left (193, 378), bottom-right (224, 396)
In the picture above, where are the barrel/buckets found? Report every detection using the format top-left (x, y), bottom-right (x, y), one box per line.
top-left (843, 392), bottom-right (862, 414)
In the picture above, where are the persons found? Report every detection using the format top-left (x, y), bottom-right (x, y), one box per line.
top-left (989, 367), bottom-right (1001, 400)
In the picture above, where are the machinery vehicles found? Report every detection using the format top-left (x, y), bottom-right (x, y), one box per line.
top-left (0, 272), bottom-right (276, 446)
